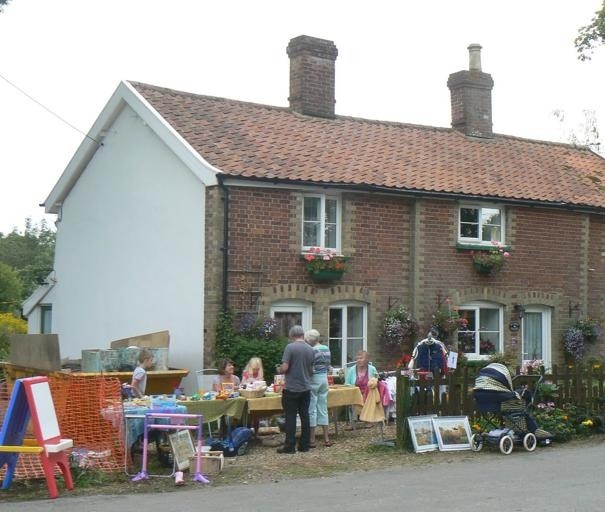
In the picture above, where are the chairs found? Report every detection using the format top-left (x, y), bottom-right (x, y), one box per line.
top-left (345, 361), bottom-right (372, 426)
top-left (195, 368), bottom-right (224, 430)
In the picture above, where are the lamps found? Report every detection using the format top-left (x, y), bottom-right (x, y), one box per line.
top-left (509, 303), bottom-right (525, 331)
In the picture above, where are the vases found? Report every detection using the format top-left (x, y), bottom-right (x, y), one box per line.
top-left (474, 262), bottom-right (493, 275)
top-left (309, 269), bottom-right (344, 283)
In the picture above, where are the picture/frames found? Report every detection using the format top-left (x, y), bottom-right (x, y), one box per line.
top-left (169, 430), bottom-right (196, 471)
top-left (432, 415), bottom-right (474, 452)
top-left (407, 414), bottom-right (439, 453)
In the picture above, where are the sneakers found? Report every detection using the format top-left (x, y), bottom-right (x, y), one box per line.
top-left (277, 447), bottom-right (295, 453)
top-left (298, 446), bottom-right (308, 452)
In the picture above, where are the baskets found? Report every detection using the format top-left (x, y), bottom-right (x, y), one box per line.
top-left (238, 385), bottom-right (266, 399)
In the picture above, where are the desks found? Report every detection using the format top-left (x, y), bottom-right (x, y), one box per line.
top-left (238, 383), bottom-right (365, 438)
top-left (101, 403), bottom-right (187, 465)
top-left (170, 396), bottom-right (248, 439)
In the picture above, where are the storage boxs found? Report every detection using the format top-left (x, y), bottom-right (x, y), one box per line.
top-left (188, 451), bottom-right (224, 476)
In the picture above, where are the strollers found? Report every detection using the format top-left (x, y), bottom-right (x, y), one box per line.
top-left (465, 360), bottom-right (546, 456)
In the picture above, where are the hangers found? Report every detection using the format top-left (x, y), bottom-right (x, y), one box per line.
top-left (425, 334), bottom-right (433, 345)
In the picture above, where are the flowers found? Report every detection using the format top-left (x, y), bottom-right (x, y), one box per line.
top-left (302, 245), bottom-right (354, 273)
top-left (470, 240), bottom-right (511, 272)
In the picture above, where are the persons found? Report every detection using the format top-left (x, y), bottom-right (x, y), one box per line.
top-left (213, 358), bottom-right (240, 436)
top-left (345, 350), bottom-right (379, 429)
top-left (277, 325), bottom-right (315, 453)
top-left (461, 224), bottom-right (474, 236)
top-left (304, 329), bottom-right (333, 447)
top-left (130, 349), bottom-right (153, 398)
top-left (242, 357), bottom-right (264, 439)
